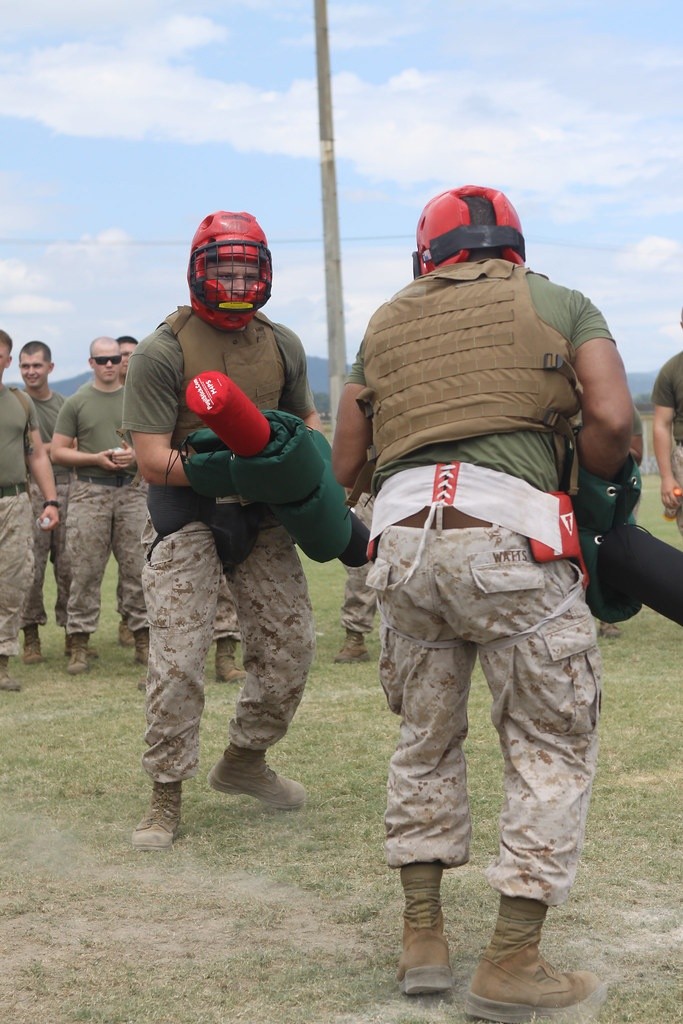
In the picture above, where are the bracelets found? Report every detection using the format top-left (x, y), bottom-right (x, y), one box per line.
top-left (43, 501), bottom-right (61, 510)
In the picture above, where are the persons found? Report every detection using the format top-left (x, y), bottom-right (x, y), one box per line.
top-left (331, 184), bottom-right (633, 1024)
top-left (50, 337), bottom-right (150, 674)
top-left (0, 330), bottom-right (59, 691)
top-left (599, 398), bottom-right (643, 639)
top-left (333, 490), bottom-right (376, 664)
top-left (651, 307), bottom-right (683, 536)
top-left (122, 211), bottom-right (324, 850)
top-left (20, 341), bottom-right (99, 665)
top-left (213, 562), bottom-right (247, 684)
top-left (115, 336), bottom-right (138, 380)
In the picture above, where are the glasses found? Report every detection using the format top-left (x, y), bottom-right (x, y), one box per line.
top-left (90, 355), bottom-right (122, 365)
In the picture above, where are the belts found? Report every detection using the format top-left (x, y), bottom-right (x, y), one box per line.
top-left (26, 473), bottom-right (71, 483)
top-left (0, 481), bottom-right (29, 497)
top-left (392, 507), bottom-right (502, 529)
top-left (77, 475), bottom-right (136, 487)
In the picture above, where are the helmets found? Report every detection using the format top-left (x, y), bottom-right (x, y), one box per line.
top-left (187, 210), bottom-right (272, 330)
top-left (412, 184), bottom-right (526, 280)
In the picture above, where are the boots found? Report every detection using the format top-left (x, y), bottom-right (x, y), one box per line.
top-left (466, 893), bottom-right (603, 1024)
top-left (334, 628), bottom-right (370, 663)
top-left (64, 626), bottom-right (99, 659)
top-left (395, 861), bottom-right (453, 993)
top-left (208, 741), bottom-right (307, 808)
top-left (0, 654), bottom-right (21, 691)
top-left (599, 621), bottom-right (621, 637)
top-left (216, 638), bottom-right (248, 683)
top-left (68, 632), bottom-right (89, 675)
top-left (23, 623), bottom-right (43, 664)
top-left (119, 614), bottom-right (134, 648)
top-left (132, 781), bottom-right (182, 851)
top-left (134, 627), bottom-right (150, 667)
